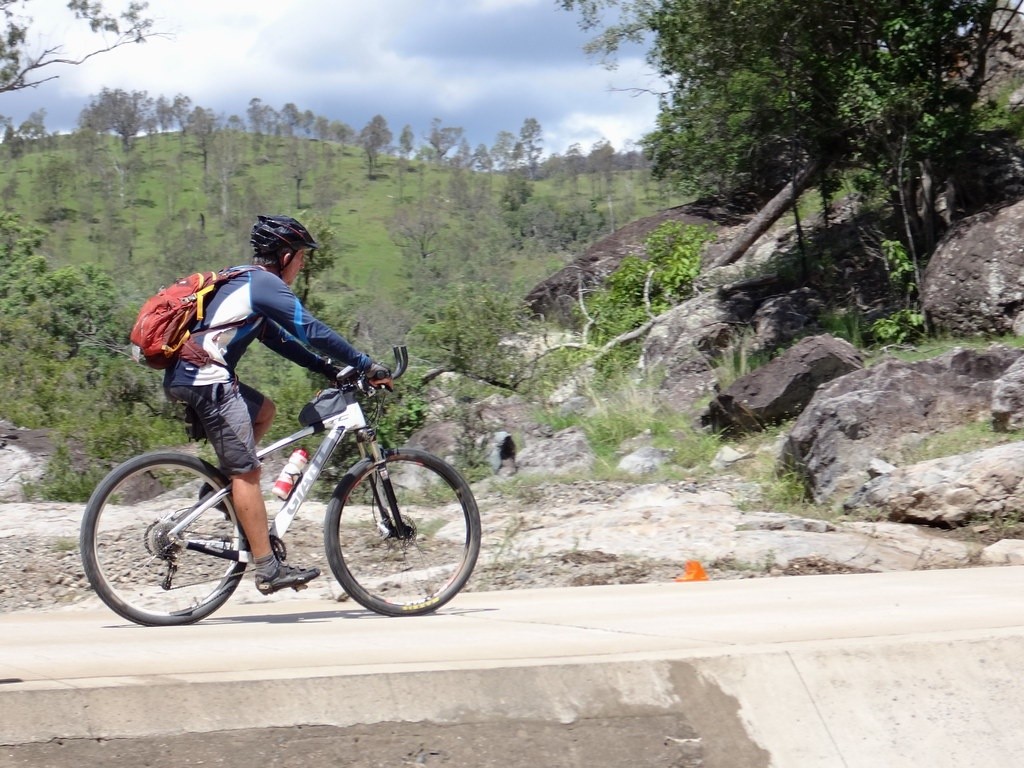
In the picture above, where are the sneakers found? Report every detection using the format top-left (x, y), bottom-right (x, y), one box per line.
top-left (254, 562), bottom-right (321, 596)
top-left (198, 482), bottom-right (231, 520)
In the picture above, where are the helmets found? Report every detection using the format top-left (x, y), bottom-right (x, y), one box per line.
top-left (250, 214), bottom-right (318, 265)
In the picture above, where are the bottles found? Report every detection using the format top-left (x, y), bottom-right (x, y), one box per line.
top-left (270, 447), bottom-right (310, 500)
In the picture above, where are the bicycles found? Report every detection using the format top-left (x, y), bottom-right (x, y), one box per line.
top-left (80, 344), bottom-right (481, 628)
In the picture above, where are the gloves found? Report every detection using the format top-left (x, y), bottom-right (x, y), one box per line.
top-left (364, 362), bottom-right (394, 393)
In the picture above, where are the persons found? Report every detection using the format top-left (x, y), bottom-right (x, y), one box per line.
top-left (162, 216), bottom-right (394, 596)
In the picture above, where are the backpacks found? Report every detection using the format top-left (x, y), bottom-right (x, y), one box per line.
top-left (130, 266), bottom-right (268, 370)
